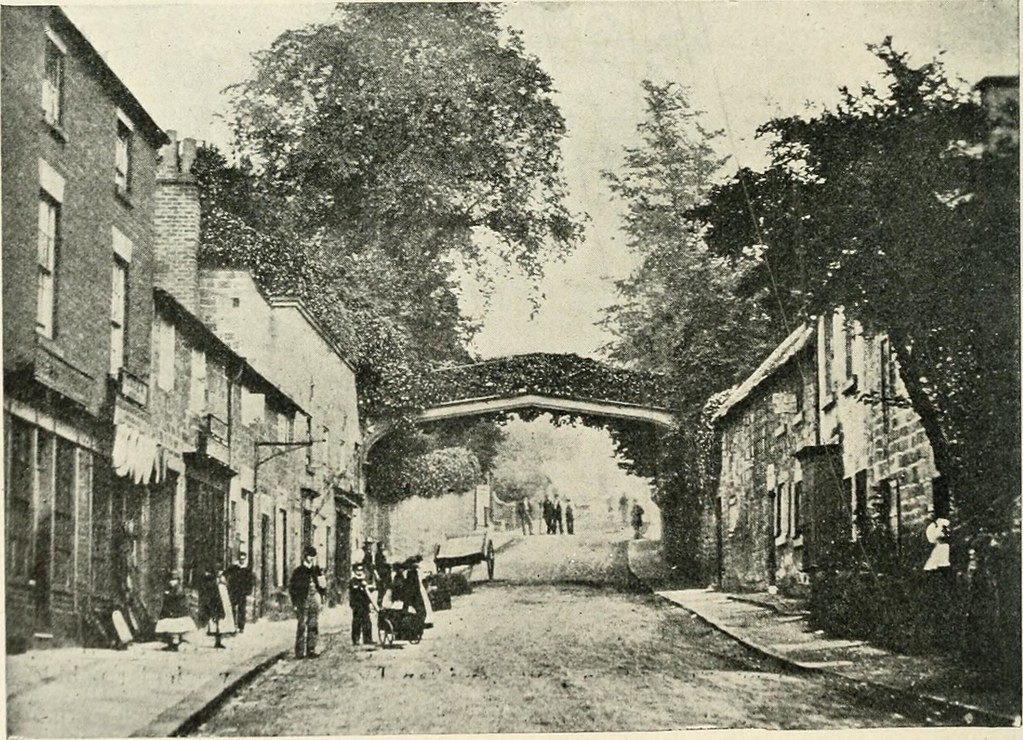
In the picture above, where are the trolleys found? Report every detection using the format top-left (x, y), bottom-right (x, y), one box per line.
top-left (365, 583), bottom-right (425, 649)
top-left (432, 529), bottom-right (495, 581)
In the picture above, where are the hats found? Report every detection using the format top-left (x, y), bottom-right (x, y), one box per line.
top-left (352, 564), bottom-right (366, 571)
top-left (362, 537), bottom-right (374, 545)
top-left (303, 545), bottom-right (317, 558)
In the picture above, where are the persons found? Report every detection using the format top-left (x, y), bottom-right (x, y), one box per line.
top-left (288, 546), bottom-right (326, 660)
top-left (391, 554), bottom-right (427, 624)
top-left (155, 551), bottom-right (253, 652)
top-left (518, 493), bottom-right (574, 535)
top-left (629, 499), bottom-right (645, 540)
top-left (620, 491), bottom-right (629, 520)
top-left (347, 563), bottom-right (378, 645)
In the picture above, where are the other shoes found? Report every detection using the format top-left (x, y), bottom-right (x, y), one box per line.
top-left (307, 650), bottom-right (320, 658)
